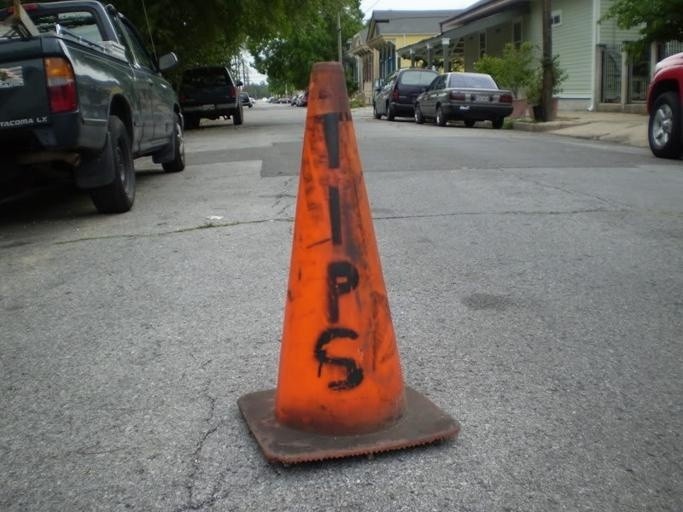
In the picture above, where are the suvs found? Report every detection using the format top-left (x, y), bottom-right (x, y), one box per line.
top-left (177, 66), bottom-right (245, 129)
top-left (647, 50), bottom-right (683, 164)
top-left (371, 67), bottom-right (441, 121)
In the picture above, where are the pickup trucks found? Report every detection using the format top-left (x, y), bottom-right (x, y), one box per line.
top-left (0, 2), bottom-right (185, 216)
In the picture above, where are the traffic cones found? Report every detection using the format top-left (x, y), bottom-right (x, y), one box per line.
top-left (236, 59), bottom-right (461, 468)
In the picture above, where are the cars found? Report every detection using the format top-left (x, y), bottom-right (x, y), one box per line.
top-left (239, 90), bottom-right (308, 108)
top-left (413, 67), bottom-right (515, 129)
